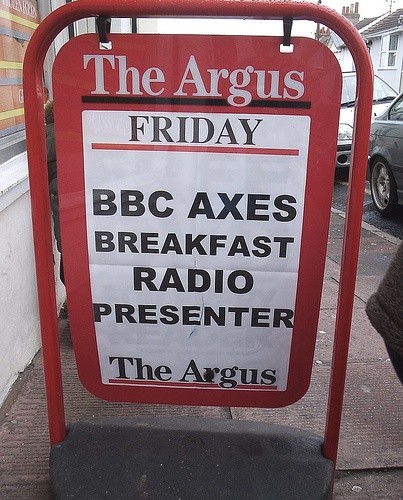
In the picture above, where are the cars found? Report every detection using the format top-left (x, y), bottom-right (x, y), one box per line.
top-left (330, 69), bottom-right (401, 169)
top-left (368, 90), bottom-right (403, 215)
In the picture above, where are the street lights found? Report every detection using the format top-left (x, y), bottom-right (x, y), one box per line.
top-left (386, 0), bottom-right (396, 14)
